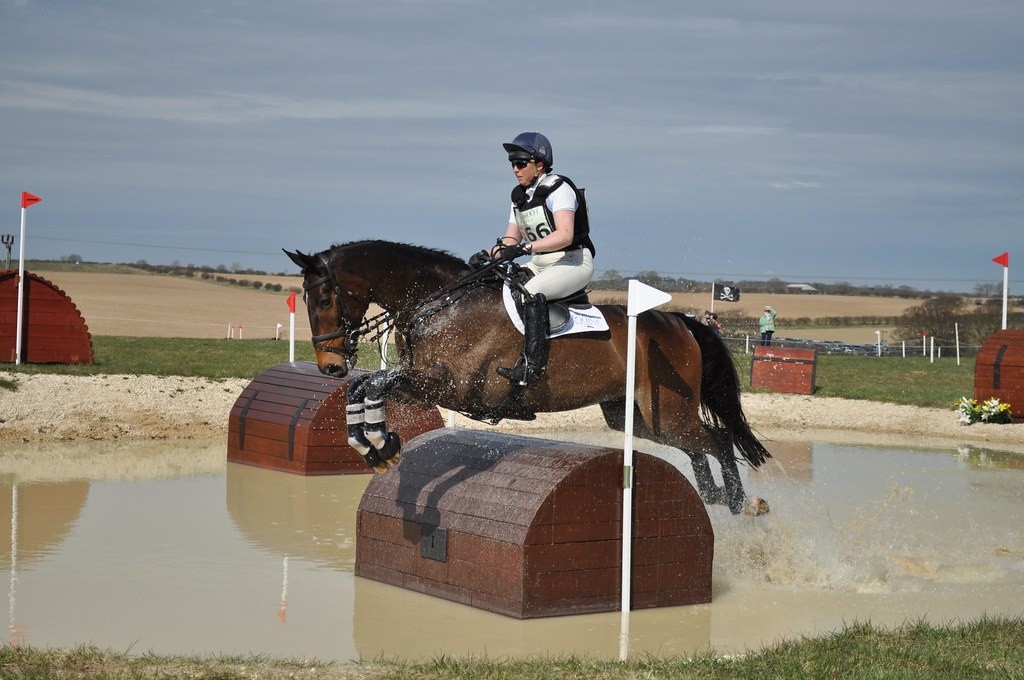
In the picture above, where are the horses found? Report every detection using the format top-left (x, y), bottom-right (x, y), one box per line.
top-left (282, 238), bottom-right (775, 518)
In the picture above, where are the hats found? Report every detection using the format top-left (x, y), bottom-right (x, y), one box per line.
top-left (502, 131), bottom-right (553, 173)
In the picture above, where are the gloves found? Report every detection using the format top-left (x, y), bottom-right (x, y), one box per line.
top-left (503, 240), bottom-right (532, 262)
top-left (468, 249), bottom-right (490, 265)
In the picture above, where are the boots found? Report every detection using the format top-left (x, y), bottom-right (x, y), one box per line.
top-left (495, 293), bottom-right (551, 384)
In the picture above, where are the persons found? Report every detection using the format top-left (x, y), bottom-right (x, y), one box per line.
top-left (472, 133), bottom-right (596, 385)
top-left (690, 311), bottom-right (721, 336)
top-left (759, 306), bottom-right (777, 348)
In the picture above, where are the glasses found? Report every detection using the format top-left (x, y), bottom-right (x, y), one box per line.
top-left (511, 159), bottom-right (527, 170)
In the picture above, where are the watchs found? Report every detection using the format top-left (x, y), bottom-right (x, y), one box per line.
top-left (524, 241), bottom-right (532, 255)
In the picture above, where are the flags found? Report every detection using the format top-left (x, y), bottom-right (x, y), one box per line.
top-left (711, 282), bottom-right (742, 303)
